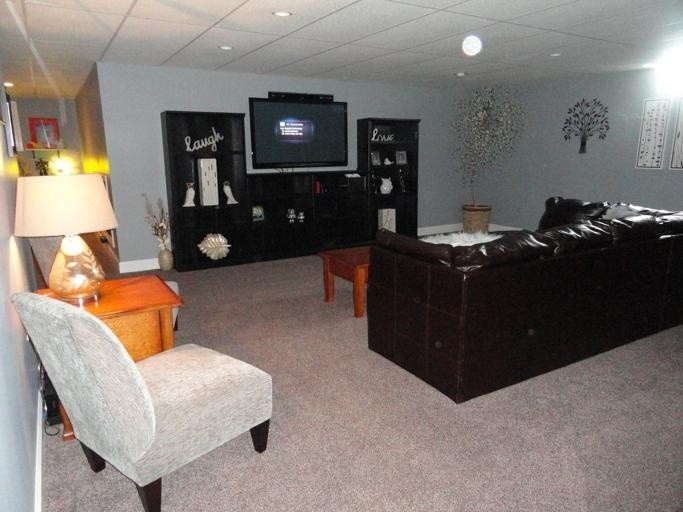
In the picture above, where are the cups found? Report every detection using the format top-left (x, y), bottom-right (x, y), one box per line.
top-left (371, 151), bottom-right (382, 166)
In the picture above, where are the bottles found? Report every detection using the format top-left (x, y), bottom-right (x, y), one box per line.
top-left (315, 181), bottom-right (320, 193)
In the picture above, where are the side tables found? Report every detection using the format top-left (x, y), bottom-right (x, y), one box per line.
top-left (37, 273), bottom-right (186, 442)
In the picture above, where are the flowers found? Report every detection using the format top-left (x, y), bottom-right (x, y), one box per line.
top-left (141, 193), bottom-right (169, 249)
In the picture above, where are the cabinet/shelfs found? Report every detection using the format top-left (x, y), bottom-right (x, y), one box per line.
top-left (246, 170), bottom-right (363, 264)
top-left (357, 118), bottom-right (421, 246)
top-left (160, 110), bottom-right (245, 272)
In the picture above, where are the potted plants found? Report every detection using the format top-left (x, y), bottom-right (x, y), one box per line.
top-left (451, 84), bottom-right (529, 234)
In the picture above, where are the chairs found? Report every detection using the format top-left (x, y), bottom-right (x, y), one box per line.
top-left (13, 292), bottom-right (274, 510)
top-left (28, 237), bottom-right (181, 335)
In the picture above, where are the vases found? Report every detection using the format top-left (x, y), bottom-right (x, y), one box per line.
top-left (158, 249), bottom-right (173, 271)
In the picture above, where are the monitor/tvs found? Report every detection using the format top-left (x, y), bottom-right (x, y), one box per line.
top-left (248, 97), bottom-right (348, 169)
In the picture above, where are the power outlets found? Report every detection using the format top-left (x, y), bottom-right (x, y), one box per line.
top-left (37, 363), bottom-right (44, 391)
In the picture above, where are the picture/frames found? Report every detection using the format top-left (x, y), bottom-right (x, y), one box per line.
top-left (28, 117), bottom-right (58, 149)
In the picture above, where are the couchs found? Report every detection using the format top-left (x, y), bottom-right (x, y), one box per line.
top-left (367, 196), bottom-right (683, 404)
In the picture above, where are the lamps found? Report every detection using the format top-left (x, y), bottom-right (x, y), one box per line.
top-left (13, 172), bottom-right (118, 305)
